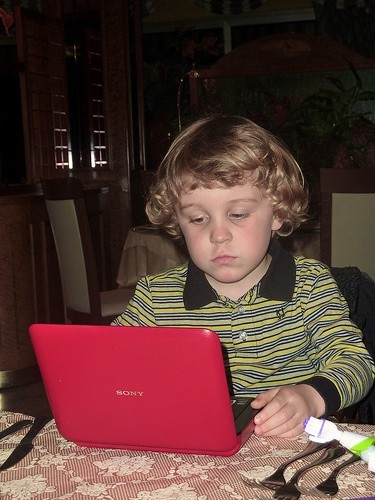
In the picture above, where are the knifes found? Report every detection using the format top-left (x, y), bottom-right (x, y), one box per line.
top-left (0, 416), bottom-right (52, 472)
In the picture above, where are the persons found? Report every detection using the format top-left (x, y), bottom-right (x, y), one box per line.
top-left (107, 114), bottom-right (375, 438)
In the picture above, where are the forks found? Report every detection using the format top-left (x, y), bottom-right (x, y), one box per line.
top-left (305, 454), bottom-right (361, 497)
top-left (269, 447), bottom-right (346, 500)
top-left (237, 439), bottom-right (338, 500)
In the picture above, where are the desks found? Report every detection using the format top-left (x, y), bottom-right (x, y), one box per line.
top-left (0, 410), bottom-right (375, 500)
top-left (115, 225), bottom-right (188, 286)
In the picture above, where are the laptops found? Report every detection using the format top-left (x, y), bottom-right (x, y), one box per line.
top-left (28, 324), bottom-right (262, 457)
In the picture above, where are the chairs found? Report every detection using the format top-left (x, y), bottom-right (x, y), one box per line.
top-left (319, 165), bottom-right (375, 282)
top-left (40, 177), bottom-right (135, 325)
